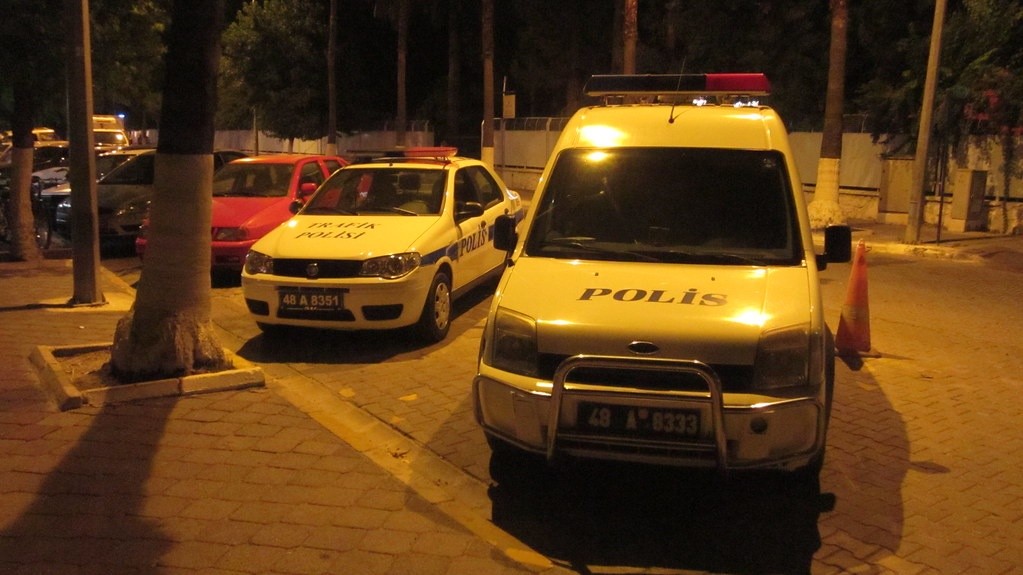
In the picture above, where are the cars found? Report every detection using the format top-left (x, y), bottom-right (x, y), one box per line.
top-left (41, 148), bottom-right (153, 215)
top-left (30, 146), bottom-right (115, 203)
top-left (57, 152), bottom-right (250, 251)
top-left (240, 143), bottom-right (525, 342)
top-left (136, 155), bottom-right (371, 280)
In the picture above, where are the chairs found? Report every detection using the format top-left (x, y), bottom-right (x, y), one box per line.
top-left (432, 179), bottom-right (465, 214)
top-left (363, 184), bottom-right (398, 212)
top-left (253, 174), bottom-right (272, 196)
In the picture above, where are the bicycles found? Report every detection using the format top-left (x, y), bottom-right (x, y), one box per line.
top-left (0, 169), bottom-right (53, 249)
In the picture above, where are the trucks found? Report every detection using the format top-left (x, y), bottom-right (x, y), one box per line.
top-left (92, 113), bottom-right (131, 154)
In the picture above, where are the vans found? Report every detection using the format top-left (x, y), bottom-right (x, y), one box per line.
top-left (471, 61), bottom-right (853, 520)
top-left (0, 126), bottom-right (70, 161)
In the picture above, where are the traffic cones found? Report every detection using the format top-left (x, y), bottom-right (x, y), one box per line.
top-left (832, 238), bottom-right (882, 358)
